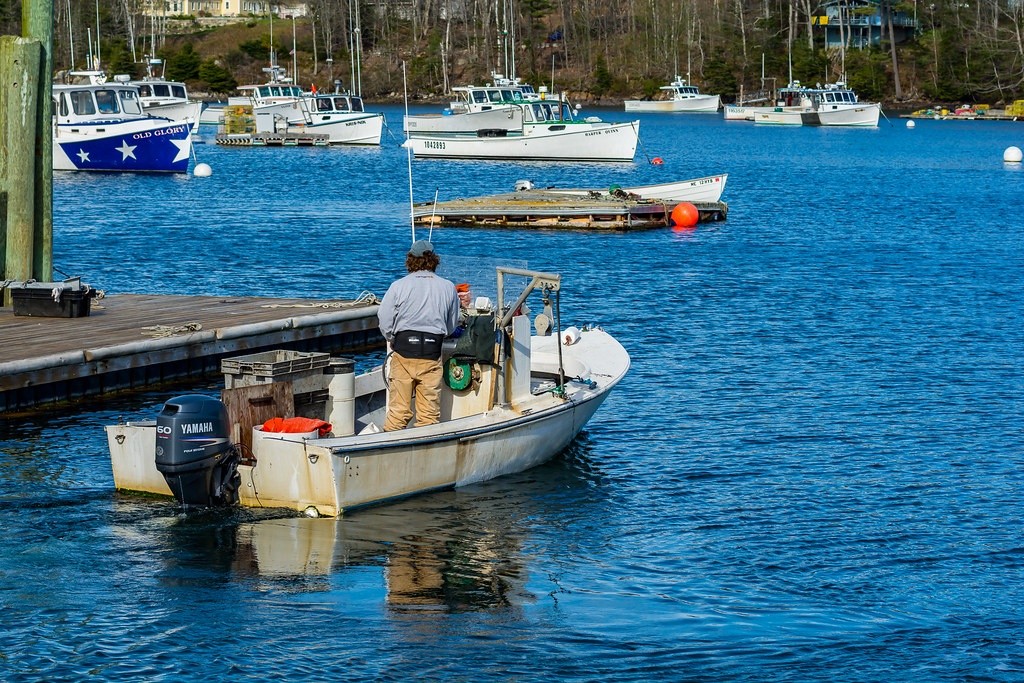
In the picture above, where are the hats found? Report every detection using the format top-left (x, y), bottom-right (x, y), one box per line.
top-left (409, 239), bottom-right (435, 257)
top-left (455, 284), bottom-right (470, 295)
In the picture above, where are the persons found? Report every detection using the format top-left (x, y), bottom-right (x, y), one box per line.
top-left (378, 240), bottom-right (461, 432)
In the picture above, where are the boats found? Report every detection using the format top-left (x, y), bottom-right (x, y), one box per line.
top-left (724, 80), bottom-right (882, 127)
top-left (402, 29), bottom-right (566, 133)
top-left (52, 71), bottom-right (196, 174)
top-left (410, 120), bottom-right (640, 162)
top-left (111, 54), bottom-right (210, 135)
top-left (515, 173), bottom-right (729, 203)
top-left (103, 265), bottom-right (632, 519)
top-left (200, 16), bottom-right (384, 145)
top-left (624, 75), bottom-right (720, 113)
